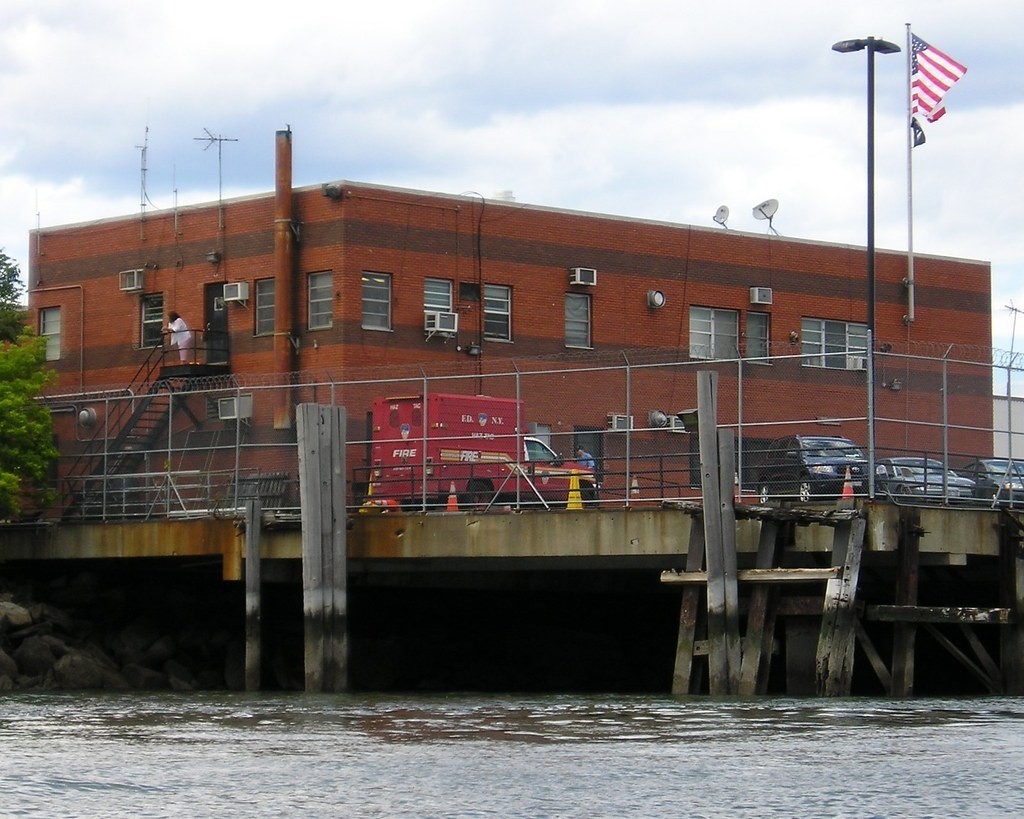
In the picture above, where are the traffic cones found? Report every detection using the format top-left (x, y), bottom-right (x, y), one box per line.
top-left (629, 474), bottom-right (643, 507)
top-left (371, 499), bottom-right (400, 512)
top-left (840, 465), bottom-right (856, 500)
top-left (566, 468), bottom-right (584, 510)
top-left (446, 480), bottom-right (459, 512)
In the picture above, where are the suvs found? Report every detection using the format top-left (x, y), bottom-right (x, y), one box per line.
top-left (757, 433), bottom-right (880, 505)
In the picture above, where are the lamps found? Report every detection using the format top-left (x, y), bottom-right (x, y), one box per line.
top-left (207, 251), bottom-right (219, 262)
top-left (467, 343), bottom-right (483, 355)
top-left (890, 382), bottom-right (902, 390)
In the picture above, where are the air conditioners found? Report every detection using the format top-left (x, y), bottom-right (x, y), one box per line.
top-left (424, 311), bottom-right (458, 332)
top-left (223, 282), bottom-right (249, 301)
top-left (749, 287), bottom-right (773, 305)
top-left (218, 397), bottom-right (252, 420)
top-left (569, 267), bottom-right (596, 285)
top-left (606, 415), bottom-right (634, 430)
top-left (667, 416), bottom-right (690, 435)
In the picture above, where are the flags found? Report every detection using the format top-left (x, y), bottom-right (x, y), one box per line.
top-left (909, 32), bottom-right (968, 124)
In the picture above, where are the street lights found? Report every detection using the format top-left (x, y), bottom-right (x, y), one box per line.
top-left (830, 35), bottom-right (904, 500)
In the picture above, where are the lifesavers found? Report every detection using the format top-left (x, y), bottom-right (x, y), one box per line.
top-left (360, 499), bottom-right (402, 514)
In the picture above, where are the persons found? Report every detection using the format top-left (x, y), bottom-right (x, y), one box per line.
top-left (162, 310), bottom-right (192, 365)
top-left (575, 445), bottom-right (595, 469)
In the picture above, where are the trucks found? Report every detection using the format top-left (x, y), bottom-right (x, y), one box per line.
top-left (370, 392), bottom-right (598, 512)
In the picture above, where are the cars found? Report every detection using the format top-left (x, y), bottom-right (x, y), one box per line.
top-left (875, 456), bottom-right (977, 506)
top-left (955, 458), bottom-right (1024, 509)
